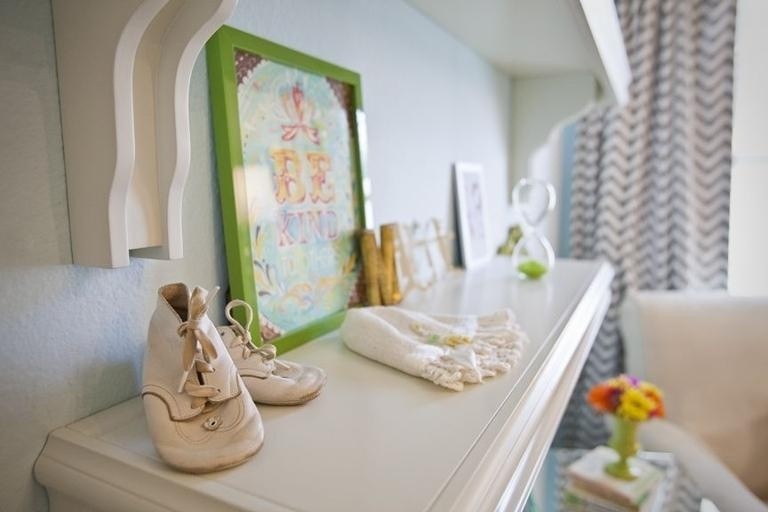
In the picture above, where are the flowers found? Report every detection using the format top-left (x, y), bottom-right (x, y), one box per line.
top-left (584, 370), bottom-right (667, 427)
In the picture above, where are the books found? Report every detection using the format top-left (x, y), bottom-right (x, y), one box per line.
top-left (559, 445), bottom-right (680, 512)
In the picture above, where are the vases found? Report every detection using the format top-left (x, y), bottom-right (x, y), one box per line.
top-left (602, 418), bottom-right (646, 482)
top-left (510, 177), bottom-right (556, 282)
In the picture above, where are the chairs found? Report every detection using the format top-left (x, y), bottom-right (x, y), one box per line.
top-left (618, 292), bottom-right (767, 504)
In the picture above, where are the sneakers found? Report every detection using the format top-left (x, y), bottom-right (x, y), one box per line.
top-left (138, 281), bottom-right (328, 477)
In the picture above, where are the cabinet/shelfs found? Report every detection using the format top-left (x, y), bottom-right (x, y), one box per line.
top-left (33, 0), bottom-right (633, 512)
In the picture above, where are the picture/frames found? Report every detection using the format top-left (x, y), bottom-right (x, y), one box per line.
top-left (205, 25), bottom-right (375, 357)
top-left (452, 162), bottom-right (506, 272)
